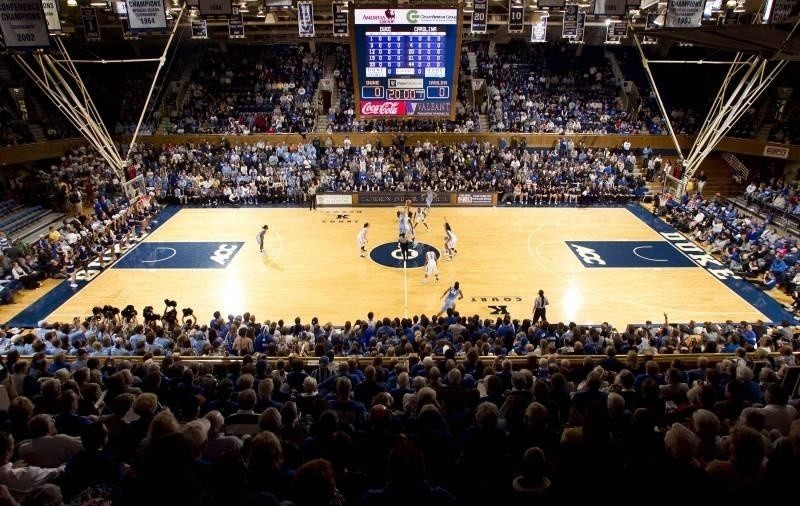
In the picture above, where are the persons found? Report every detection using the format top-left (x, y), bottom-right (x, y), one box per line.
top-left (436, 282), bottom-right (464, 317)
top-left (2, 189), bottom-right (161, 289)
top-left (358, 200), bottom-right (458, 287)
top-left (0, 306), bottom-right (800, 504)
top-left (532, 290), bottom-right (549, 324)
top-left (662, 169), bottom-right (800, 296)
top-left (256, 225), bottom-right (268, 253)
top-left (0, 36), bottom-right (791, 210)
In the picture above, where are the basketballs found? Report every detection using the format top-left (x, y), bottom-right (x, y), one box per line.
top-left (407, 199), bottom-right (412, 204)
